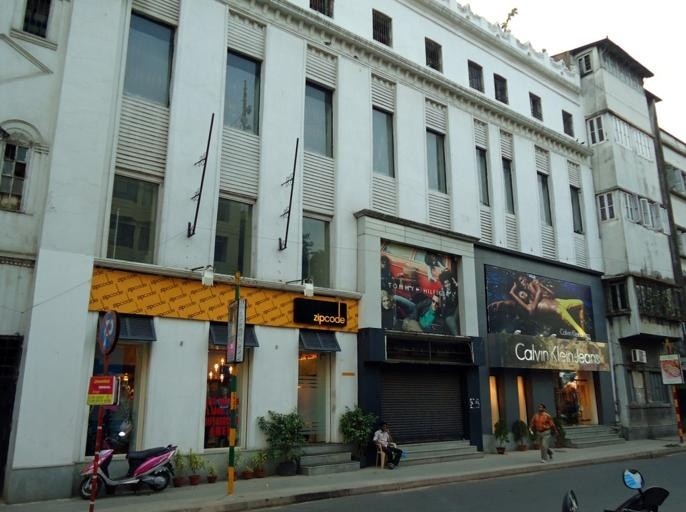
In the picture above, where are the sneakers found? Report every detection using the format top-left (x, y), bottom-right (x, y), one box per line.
top-left (550, 451), bottom-right (553, 459)
top-left (388, 462), bottom-right (395, 469)
top-left (541, 460), bottom-right (547, 463)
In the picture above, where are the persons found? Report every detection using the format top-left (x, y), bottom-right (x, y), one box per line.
top-left (518, 275), bottom-right (555, 317)
top-left (529, 403), bottom-right (561, 462)
top-left (373, 422), bottom-right (403, 468)
top-left (509, 281), bottom-right (587, 338)
top-left (381, 251), bottom-right (459, 333)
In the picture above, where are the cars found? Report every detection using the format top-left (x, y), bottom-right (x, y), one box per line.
top-left (380, 238), bottom-right (454, 304)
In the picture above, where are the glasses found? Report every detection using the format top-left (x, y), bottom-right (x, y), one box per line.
top-left (433, 300), bottom-right (439, 305)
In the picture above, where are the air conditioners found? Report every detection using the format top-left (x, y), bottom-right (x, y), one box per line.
top-left (631, 349), bottom-right (647, 364)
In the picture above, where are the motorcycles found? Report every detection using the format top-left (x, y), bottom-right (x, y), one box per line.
top-left (555, 468), bottom-right (671, 512)
top-left (77, 426), bottom-right (181, 499)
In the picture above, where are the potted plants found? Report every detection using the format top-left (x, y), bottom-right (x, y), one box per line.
top-left (553, 413), bottom-right (566, 448)
top-left (339, 400), bottom-right (379, 468)
top-left (494, 419), bottom-right (540, 454)
top-left (226, 447), bottom-right (269, 481)
top-left (256, 405), bottom-right (307, 476)
top-left (171, 447), bottom-right (218, 487)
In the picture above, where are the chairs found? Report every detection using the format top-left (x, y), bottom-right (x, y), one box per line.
top-left (376, 442), bottom-right (397, 468)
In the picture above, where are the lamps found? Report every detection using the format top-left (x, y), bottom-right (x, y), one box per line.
top-left (191, 264), bottom-right (216, 287)
top-left (286, 276), bottom-right (314, 297)
